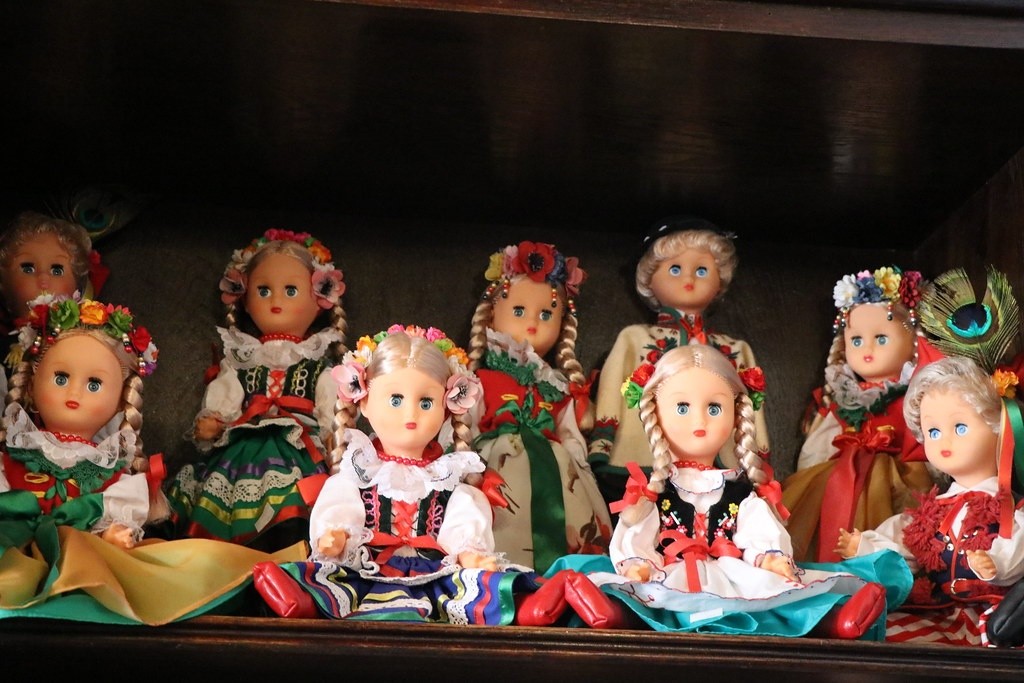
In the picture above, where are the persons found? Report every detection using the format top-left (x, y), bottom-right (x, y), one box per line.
top-left (254, 324), bottom-right (574, 626)
top-left (0, 290), bottom-right (310, 626)
top-left (589, 219), bottom-right (770, 529)
top-left (160, 229), bottom-right (348, 550)
top-left (781, 267), bottom-right (944, 562)
top-left (832, 270), bottom-right (1024, 648)
top-left (0, 188), bottom-right (133, 417)
top-left (543, 343), bottom-right (914, 642)
top-left (438, 240), bottom-right (613, 576)
